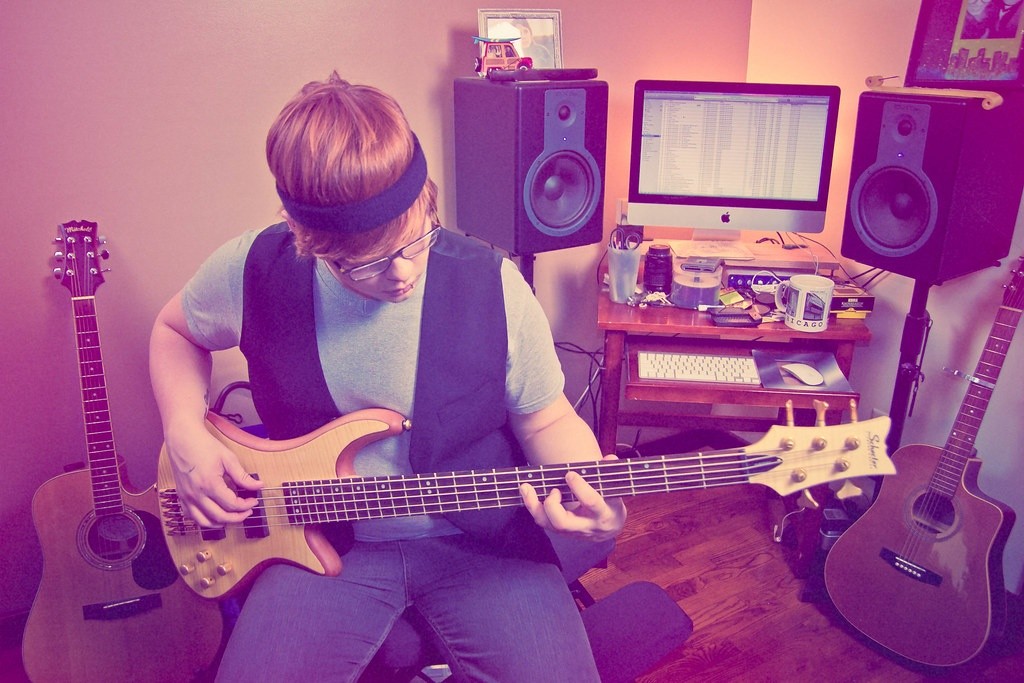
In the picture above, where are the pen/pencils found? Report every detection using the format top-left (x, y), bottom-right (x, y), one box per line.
top-left (647, 302), bottom-right (698, 310)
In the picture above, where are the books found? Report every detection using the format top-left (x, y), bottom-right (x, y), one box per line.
top-left (667, 240), bottom-right (756, 261)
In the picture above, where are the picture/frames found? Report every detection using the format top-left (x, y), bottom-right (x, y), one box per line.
top-left (477, 9), bottom-right (562, 78)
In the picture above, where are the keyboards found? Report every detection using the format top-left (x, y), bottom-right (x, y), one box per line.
top-left (638, 350), bottom-right (762, 385)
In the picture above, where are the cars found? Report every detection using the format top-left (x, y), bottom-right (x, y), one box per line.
top-left (473, 41), bottom-right (533, 72)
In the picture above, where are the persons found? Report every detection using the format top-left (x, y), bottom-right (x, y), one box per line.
top-left (149, 68), bottom-right (627, 683)
top-left (962, 0), bottom-right (1021, 38)
top-left (510, 18), bottom-right (555, 68)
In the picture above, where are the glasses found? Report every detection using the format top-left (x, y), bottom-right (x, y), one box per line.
top-left (332, 199), bottom-right (443, 282)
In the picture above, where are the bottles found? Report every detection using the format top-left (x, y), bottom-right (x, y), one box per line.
top-left (642, 243), bottom-right (674, 296)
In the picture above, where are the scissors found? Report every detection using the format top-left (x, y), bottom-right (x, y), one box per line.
top-left (609, 226), bottom-right (642, 250)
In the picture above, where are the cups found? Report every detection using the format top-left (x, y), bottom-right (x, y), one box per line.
top-left (774, 274), bottom-right (836, 333)
top-left (608, 242), bottom-right (640, 306)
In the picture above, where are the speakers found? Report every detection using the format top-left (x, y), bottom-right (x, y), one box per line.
top-left (454, 76), bottom-right (609, 259)
top-left (842, 91), bottom-right (1024, 288)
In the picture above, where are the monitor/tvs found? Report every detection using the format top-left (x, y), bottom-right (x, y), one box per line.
top-left (627, 80), bottom-right (841, 261)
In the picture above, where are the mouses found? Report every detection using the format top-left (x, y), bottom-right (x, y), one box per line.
top-left (781, 364), bottom-right (824, 386)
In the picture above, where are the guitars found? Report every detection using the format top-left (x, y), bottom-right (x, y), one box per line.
top-left (821, 256), bottom-right (1024, 671)
top-left (155, 403), bottom-right (897, 603)
top-left (20, 213), bottom-right (225, 683)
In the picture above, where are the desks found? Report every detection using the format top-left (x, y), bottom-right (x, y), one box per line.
top-left (596, 230), bottom-right (869, 580)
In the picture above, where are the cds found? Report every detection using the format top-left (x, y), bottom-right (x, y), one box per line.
top-left (674, 276), bottom-right (720, 289)
